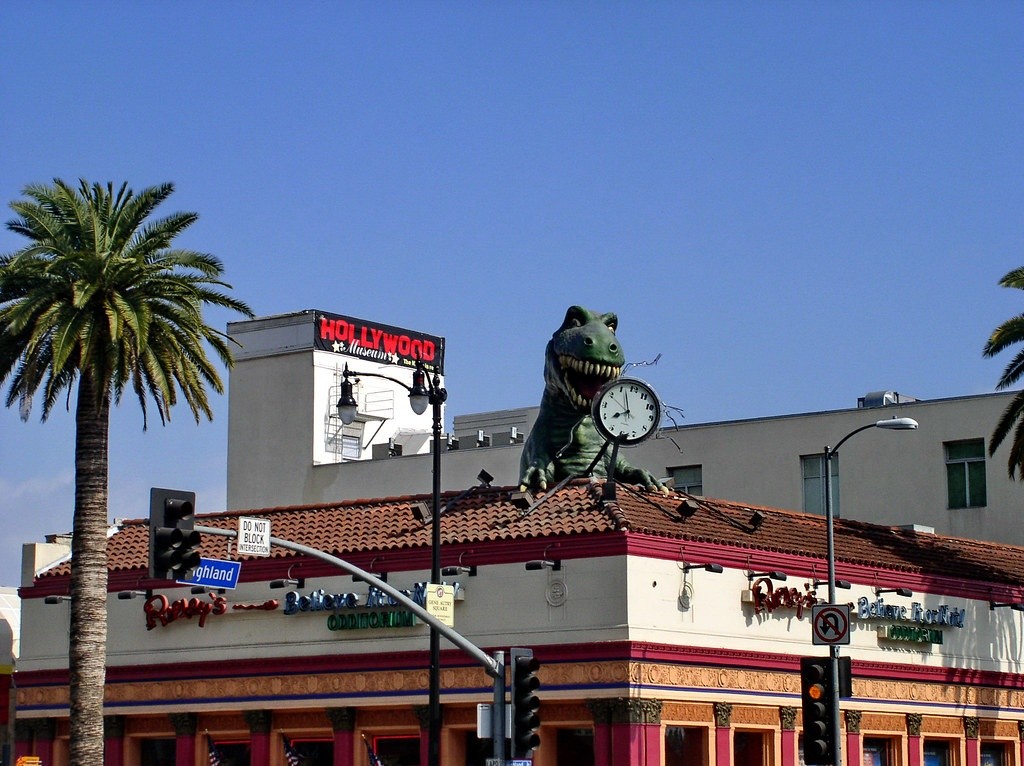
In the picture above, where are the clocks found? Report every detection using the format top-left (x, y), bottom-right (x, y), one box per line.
top-left (590, 376), bottom-right (665, 449)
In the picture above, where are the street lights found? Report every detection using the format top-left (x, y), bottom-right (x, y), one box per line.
top-left (820, 418), bottom-right (918, 766)
top-left (335, 363), bottom-right (448, 766)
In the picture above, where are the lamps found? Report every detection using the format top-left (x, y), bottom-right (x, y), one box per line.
top-left (683, 561), bottom-right (723, 574)
top-left (748, 510), bottom-right (767, 532)
top-left (441, 565), bottom-right (478, 577)
top-left (676, 501), bottom-right (700, 525)
top-left (875, 586), bottom-right (912, 598)
top-left (748, 569), bottom-right (787, 582)
top-left (511, 490), bottom-right (534, 510)
top-left (411, 501), bottom-right (431, 525)
top-left (658, 476), bottom-right (675, 492)
top-left (813, 577), bottom-right (852, 590)
top-left (990, 600), bottom-right (1024, 612)
top-left (525, 559), bottom-right (562, 571)
top-left (44, 595), bottom-right (72, 605)
top-left (353, 571), bottom-right (387, 583)
top-left (270, 578), bottom-right (305, 589)
top-left (476, 468), bottom-right (494, 487)
top-left (191, 586), bottom-right (226, 595)
top-left (118, 590), bottom-right (152, 600)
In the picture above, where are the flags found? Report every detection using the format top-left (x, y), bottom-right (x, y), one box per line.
top-left (283, 744), bottom-right (306, 766)
top-left (366, 746), bottom-right (382, 766)
top-left (207, 739), bottom-right (220, 766)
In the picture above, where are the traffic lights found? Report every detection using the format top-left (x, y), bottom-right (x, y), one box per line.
top-left (800, 656), bottom-right (835, 766)
top-left (511, 648), bottom-right (541, 758)
top-left (149, 488), bottom-right (202, 581)
